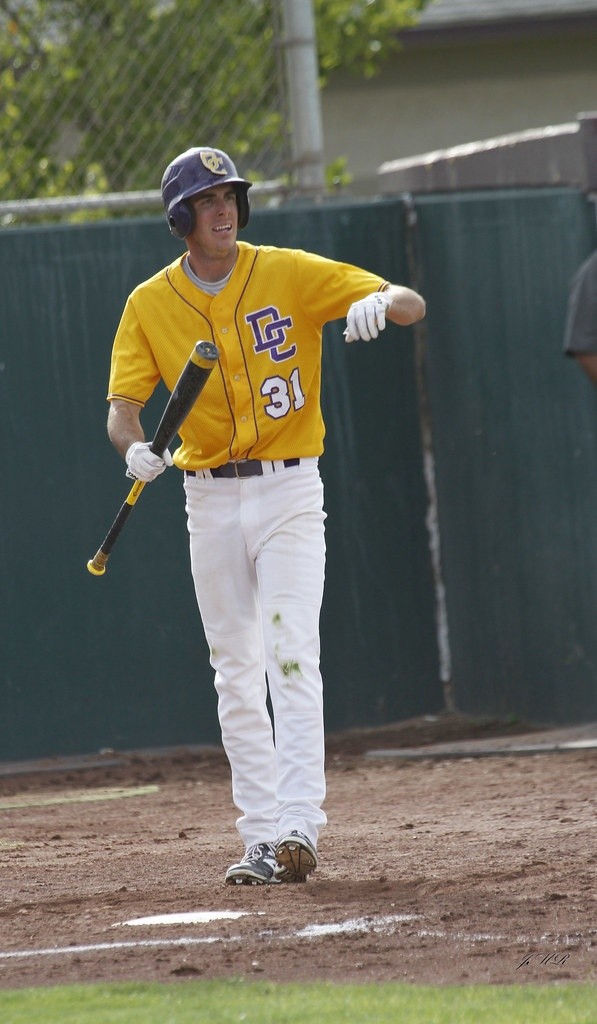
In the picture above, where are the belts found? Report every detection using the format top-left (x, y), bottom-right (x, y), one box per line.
top-left (186, 457), bottom-right (299, 477)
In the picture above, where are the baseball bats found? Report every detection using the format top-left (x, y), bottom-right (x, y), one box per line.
top-left (86, 340), bottom-right (220, 577)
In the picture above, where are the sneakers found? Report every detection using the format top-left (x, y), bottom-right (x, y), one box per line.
top-left (273, 829), bottom-right (318, 886)
top-left (226, 842), bottom-right (277, 886)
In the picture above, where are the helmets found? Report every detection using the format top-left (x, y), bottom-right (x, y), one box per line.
top-left (161, 147), bottom-right (253, 240)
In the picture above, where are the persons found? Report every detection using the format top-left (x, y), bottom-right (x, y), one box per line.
top-left (107, 147), bottom-right (426, 884)
top-left (564, 250), bottom-right (597, 383)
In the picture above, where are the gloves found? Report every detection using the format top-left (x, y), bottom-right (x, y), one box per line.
top-left (124, 441), bottom-right (173, 482)
top-left (342, 292), bottom-right (391, 342)
